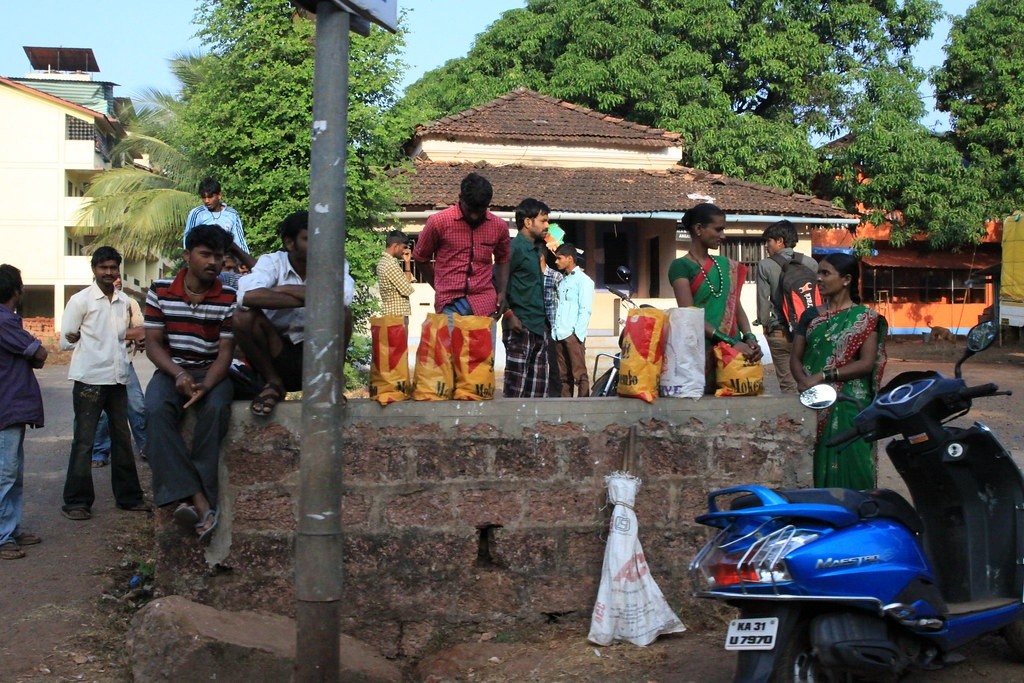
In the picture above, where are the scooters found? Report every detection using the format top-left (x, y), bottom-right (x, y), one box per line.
top-left (686, 318), bottom-right (1023, 683)
top-left (588, 265), bottom-right (659, 397)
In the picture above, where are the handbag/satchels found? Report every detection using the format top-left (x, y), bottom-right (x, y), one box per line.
top-left (659, 307), bottom-right (705, 401)
top-left (714, 341), bottom-right (764, 397)
top-left (411, 314), bottom-right (454, 402)
top-left (370, 315), bottom-right (410, 406)
top-left (450, 312), bottom-right (494, 401)
top-left (617, 308), bottom-right (666, 402)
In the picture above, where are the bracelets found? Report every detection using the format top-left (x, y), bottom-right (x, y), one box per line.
top-left (743, 333), bottom-right (756, 340)
top-left (712, 329), bottom-right (730, 344)
top-left (503, 309), bottom-right (513, 318)
top-left (405, 270), bottom-right (410, 272)
top-left (822, 368), bottom-right (840, 381)
top-left (176, 371), bottom-right (185, 379)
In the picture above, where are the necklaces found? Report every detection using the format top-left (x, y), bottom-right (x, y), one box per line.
top-left (211, 208), bottom-right (221, 219)
top-left (689, 251), bottom-right (723, 298)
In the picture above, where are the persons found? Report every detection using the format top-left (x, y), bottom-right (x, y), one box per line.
top-left (668, 203), bottom-right (762, 395)
top-left (59, 246), bottom-right (151, 518)
top-left (232, 210), bottom-right (355, 417)
top-left (493, 198), bottom-right (550, 398)
top-left (413, 172), bottom-right (511, 331)
top-left (182, 178), bottom-right (249, 289)
top-left (376, 230), bottom-right (417, 326)
top-left (142, 224), bottom-right (237, 545)
top-left (790, 253), bottom-right (889, 491)
top-left (551, 243), bottom-right (595, 397)
top-left (540, 245), bottom-right (564, 328)
top-left (0, 264), bottom-right (47, 559)
top-left (752, 221), bottom-right (818, 394)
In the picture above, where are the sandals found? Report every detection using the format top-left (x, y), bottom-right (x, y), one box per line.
top-left (251, 382), bottom-right (285, 416)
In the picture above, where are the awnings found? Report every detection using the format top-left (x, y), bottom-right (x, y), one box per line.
top-left (860, 249), bottom-right (1002, 268)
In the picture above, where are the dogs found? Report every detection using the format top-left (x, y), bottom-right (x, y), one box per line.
top-left (927, 321), bottom-right (954, 344)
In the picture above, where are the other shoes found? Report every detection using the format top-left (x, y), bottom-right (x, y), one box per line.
top-left (91, 460), bottom-right (104, 467)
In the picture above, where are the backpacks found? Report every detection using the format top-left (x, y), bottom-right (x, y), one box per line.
top-left (767, 252), bottom-right (825, 341)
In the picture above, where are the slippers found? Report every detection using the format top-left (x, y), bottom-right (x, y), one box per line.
top-left (174, 505), bottom-right (198, 527)
top-left (116, 502), bottom-right (151, 511)
top-left (0, 546), bottom-right (24, 559)
top-left (14, 533), bottom-right (41, 544)
top-left (61, 505), bottom-right (90, 520)
top-left (196, 510), bottom-right (217, 540)
